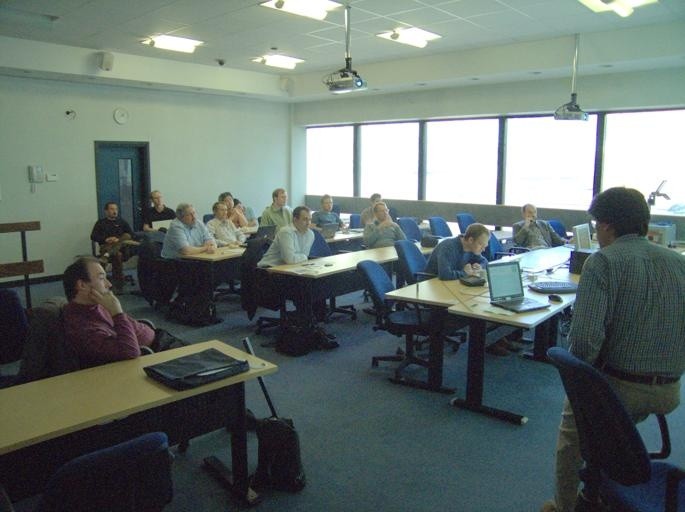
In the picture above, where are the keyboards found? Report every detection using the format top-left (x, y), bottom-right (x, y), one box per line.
top-left (530, 279), bottom-right (580, 293)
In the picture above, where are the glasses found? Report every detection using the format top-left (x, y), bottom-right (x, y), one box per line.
top-left (377, 209), bottom-right (389, 212)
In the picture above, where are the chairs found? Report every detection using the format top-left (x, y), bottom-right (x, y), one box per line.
top-left (483, 233), bottom-right (531, 262)
top-left (349, 214), bottom-right (361, 228)
top-left (41, 432), bottom-right (174, 512)
top-left (32, 295), bottom-right (191, 455)
top-left (308, 231), bottom-right (357, 324)
top-left (249, 239), bottom-right (295, 335)
top-left (356, 259), bottom-right (434, 381)
top-left (0, 287), bottom-right (40, 388)
top-left (393, 238), bottom-right (468, 353)
top-left (456, 213), bottom-right (479, 234)
top-left (429, 217), bottom-right (453, 238)
top-left (398, 217), bottom-right (423, 242)
top-left (548, 346), bottom-right (684, 512)
top-left (548, 219), bottom-right (569, 247)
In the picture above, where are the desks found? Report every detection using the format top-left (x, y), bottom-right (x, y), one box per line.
top-left (447, 265), bottom-right (582, 426)
top-left (319, 220), bottom-right (372, 248)
top-left (176, 243), bottom-right (247, 324)
top-left (1, 339), bottom-right (278, 507)
top-left (385, 247), bottom-right (574, 396)
top-left (267, 248), bottom-right (400, 330)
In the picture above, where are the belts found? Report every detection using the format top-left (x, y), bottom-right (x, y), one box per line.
top-left (601, 365), bottom-right (680, 386)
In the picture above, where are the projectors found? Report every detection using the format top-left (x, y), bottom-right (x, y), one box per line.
top-left (553, 34), bottom-right (593, 122)
top-left (321, 6), bottom-right (368, 95)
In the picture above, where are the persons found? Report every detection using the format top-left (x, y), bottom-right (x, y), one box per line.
top-left (255, 206), bottom-right (339, 348)
top-left (309, 195), bottom-right (350, 235)
top-left (91, 202), bottom-right (138, 295)
top-left (546, 188), bottom-right (685, 512)
top-left (159, 202), bottom-right (225, 325)
top-left (360, 193), bottom-right (382, 230)
top-left (206, 202), bottom-right (246, 249)
top-left (365, 201), bottom-right (407, 248)
top-left (143, 191), bottom-right (179, 236)
top-left (420, 223), bottom-right (509, 354)
top-left (234, 199), bottom-right (259, 228)
top-left (260, 189), bottom-right (294, 237)
top-left (61, 259), bottom-right (262, 434)
top-left (513, 205), bottom-right (571, 251)
top-left (219, 191), bottom-right (247, 229)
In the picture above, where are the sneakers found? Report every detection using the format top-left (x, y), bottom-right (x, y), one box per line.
top-left (485, 343), bottom-right (510, 357)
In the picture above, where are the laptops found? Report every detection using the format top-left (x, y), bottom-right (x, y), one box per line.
top-left (484, 262), bottom-right (551, 312)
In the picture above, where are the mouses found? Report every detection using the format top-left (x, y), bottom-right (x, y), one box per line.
top-left (548, 293), bottom-right (563, 302)
